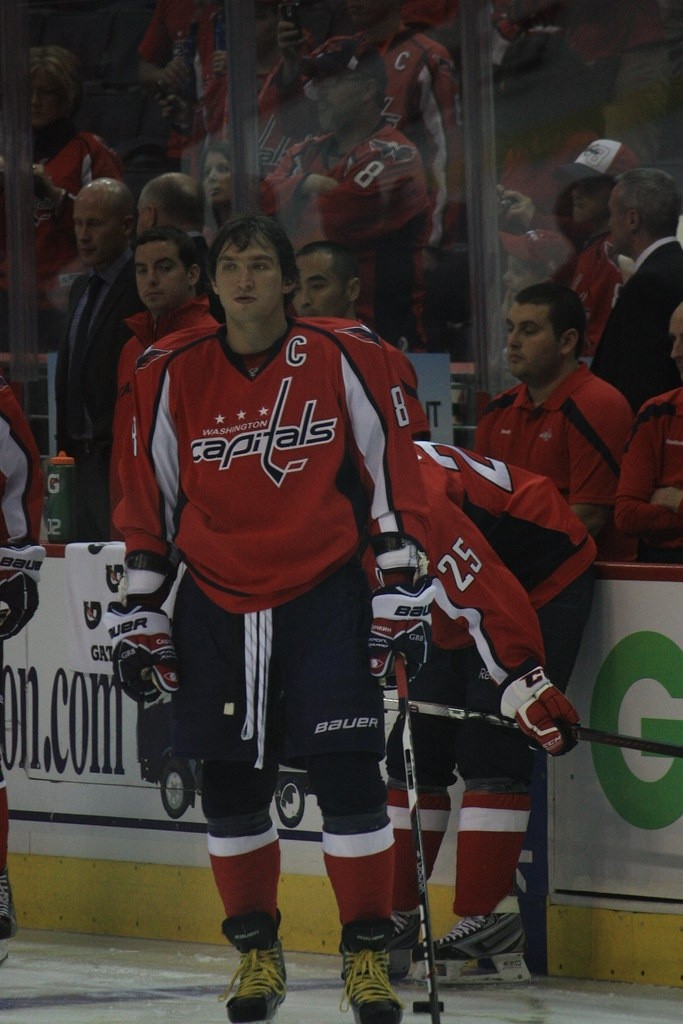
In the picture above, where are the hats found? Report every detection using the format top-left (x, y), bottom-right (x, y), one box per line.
top-left (554, 139), bottom-right (642, 193)
top-left (497, 229), bottom-right (577, 279)
top-left (296, 34), bottom-right (386, 82)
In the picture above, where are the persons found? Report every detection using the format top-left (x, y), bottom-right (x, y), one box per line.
top-left (0, 0), bottom-right (683, 582)
top-left (110, 214), bottom-right (432, 1023)
top-left (475, 282), bottom-right (634, 523)
top-left (0, 374), bottom-right (47, 965)
top-left (361, 440), bottom-right (598, 990)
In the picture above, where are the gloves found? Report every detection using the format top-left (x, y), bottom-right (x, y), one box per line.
top-left (0, 543), bottom-right (47, 643)
top-left (356, 576), bottom-right (437, 696)
top-left (103, 598), bottom-right (181, 708)
top-left (494, 661), bottom-right (585, 754)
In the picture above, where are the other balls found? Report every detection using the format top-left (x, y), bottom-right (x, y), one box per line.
top-left (413, 1001), bottom-right (445, 1014)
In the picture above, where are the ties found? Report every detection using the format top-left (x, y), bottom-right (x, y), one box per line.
top-left (71, 276), bottom-right (104, 381)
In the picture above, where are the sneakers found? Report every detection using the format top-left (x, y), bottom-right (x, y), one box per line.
top-left (0, 866), bottom-right (15, 965)
top-left (403, 905), bottom-right (534, 989)
top-left (386, 901), bottom-right (422, 979)
top-left (333, 918), bottom-right (407, 1024)
top-left (217, 903), bottom-right (287, 1024)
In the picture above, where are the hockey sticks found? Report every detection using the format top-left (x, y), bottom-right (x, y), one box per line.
top-left (382, 694), bottom-right (683, 760)
top-left (395, 640), bottom-right (441, 1024)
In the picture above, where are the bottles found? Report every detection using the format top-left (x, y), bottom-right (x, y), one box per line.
top-left (46, 451), bottom-right (76, 544)
